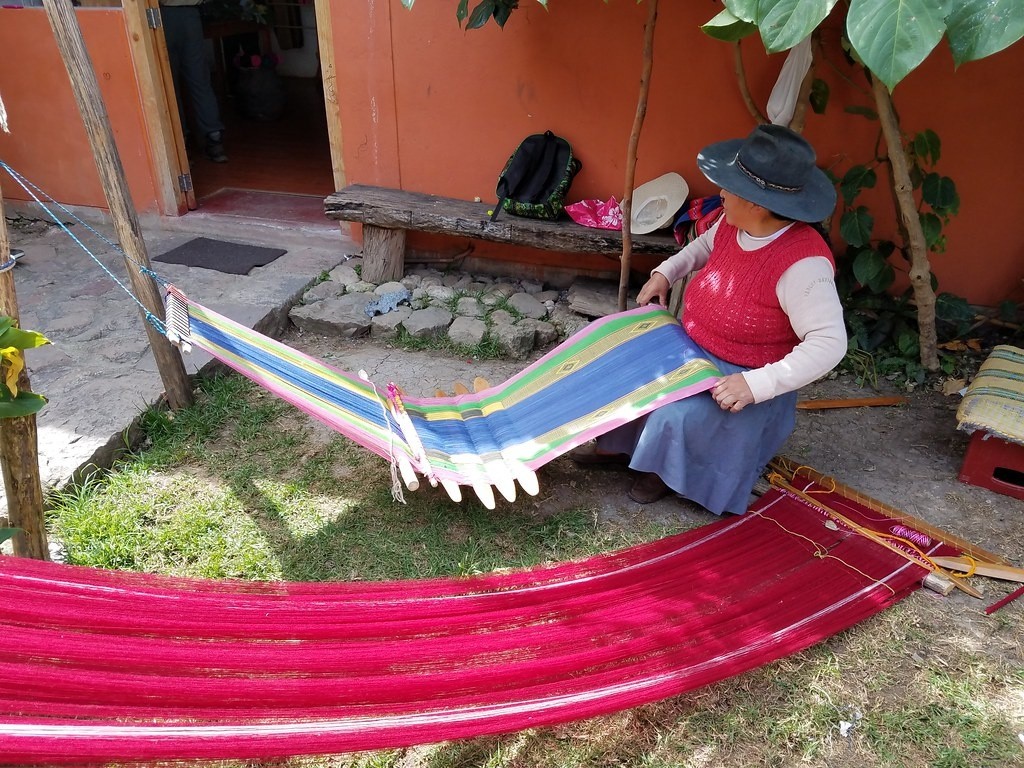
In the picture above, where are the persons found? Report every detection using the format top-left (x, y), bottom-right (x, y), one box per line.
top-left (571, 124), bottom-right (848, 516)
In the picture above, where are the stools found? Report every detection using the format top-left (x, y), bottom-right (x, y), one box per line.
top-left (957, 345), bottom-right (1024, 502)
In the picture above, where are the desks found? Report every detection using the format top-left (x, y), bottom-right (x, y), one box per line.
top-left (203, 17), bottom-right (272, 102)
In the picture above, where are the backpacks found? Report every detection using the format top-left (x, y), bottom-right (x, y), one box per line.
top-left (489, 130), bottom-right (583, 224)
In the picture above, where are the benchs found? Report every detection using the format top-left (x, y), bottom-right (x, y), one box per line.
top-left (326, 184), bottom-right (703, 323)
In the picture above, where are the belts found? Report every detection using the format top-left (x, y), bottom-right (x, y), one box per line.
top-left (164, 5), bottom-right (199, 8)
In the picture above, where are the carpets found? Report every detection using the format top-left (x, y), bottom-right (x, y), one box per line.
top-left (151, 237), bottom-right (288, 276)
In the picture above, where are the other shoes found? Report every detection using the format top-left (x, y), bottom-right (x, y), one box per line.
top-left (570, 443), bottom-right (632, 471)
top-left (11, 249), bottom-right (25, 258)
top-left (629, 472), bottom-right (673, 504)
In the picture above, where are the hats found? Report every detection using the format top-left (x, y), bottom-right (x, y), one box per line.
top-left (619, 171), bottom-right (690, 234)
top-left (696, 125), bottom-right (838, 222)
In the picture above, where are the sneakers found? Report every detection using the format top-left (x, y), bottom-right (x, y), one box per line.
top-left (201, 131), bottom-right (229, 162)
top-left (185, 144), bottom-right (194, 171)
